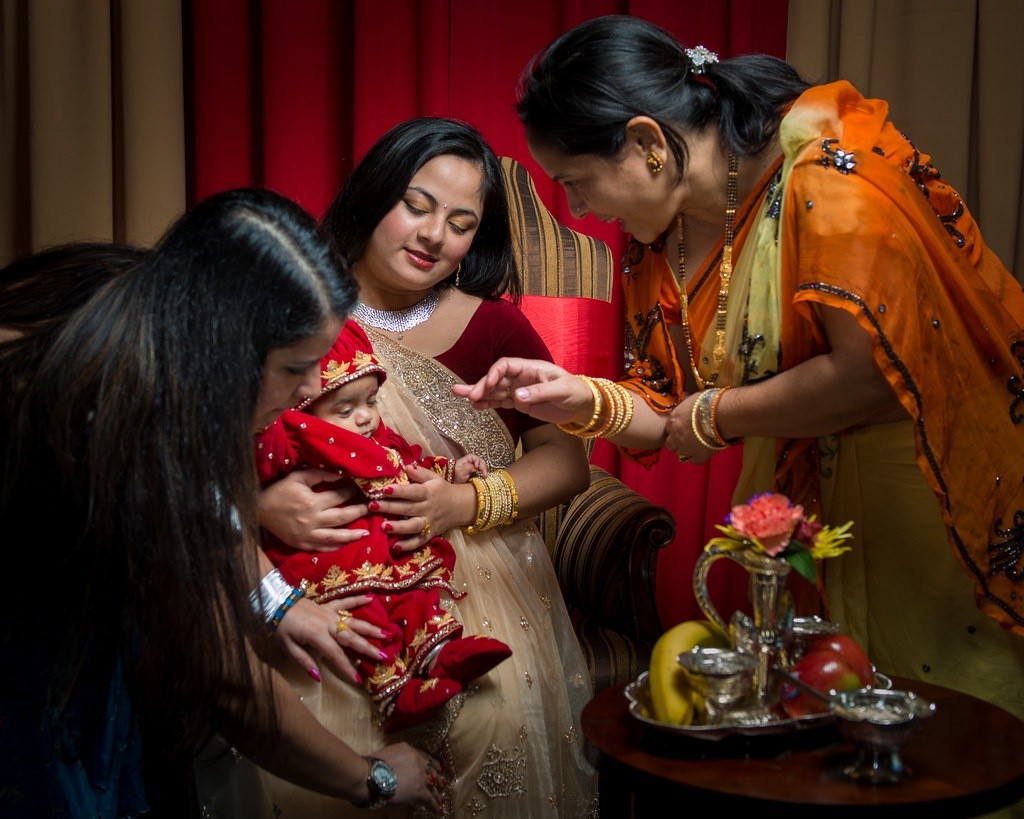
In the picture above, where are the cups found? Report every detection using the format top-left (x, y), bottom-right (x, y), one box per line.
top-left (825, 685), bottom-right (936, 787)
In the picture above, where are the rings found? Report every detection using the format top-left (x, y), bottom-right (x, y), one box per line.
top-left (680, 455), bottom-right (690, 464)
top-left (337, 610), bottom-right (353, 621)
top-left (508, 387), bottom-right (511, 396)
top-left (425, 759), bottom-right (433, 773)
top-left (335, 620), bottom-right (350, 638)
top-left (419, 518), bottom-right (431, 536)
top-left (426, 775), bottom-right (437, 787)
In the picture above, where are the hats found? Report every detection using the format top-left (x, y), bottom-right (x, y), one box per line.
top-left (290, 318), bottom-right (387, 412)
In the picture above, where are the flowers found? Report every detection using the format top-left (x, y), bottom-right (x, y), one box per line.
top-left (702, 489), bottom-right (855, 585)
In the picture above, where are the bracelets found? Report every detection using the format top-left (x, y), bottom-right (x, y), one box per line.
top-left (555, 372), bottom-right (633, 440)
top-left (247, 568), bottom-right (294, 626)
top-left (462, 469), bottom-right (518, 535)
top-left (692, 385), bottom-right (739, 451)
top-left (267, 577), bottom-right (309, 638)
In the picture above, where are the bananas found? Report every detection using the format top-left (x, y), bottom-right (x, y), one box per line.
top-left (649, 619), bottom-right (719, 726)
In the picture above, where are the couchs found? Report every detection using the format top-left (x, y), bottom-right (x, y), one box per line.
top-left (514, 443), bottom-right (684, 690)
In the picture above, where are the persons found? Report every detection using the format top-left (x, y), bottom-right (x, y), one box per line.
top-left (237, 318), bottom-right (511, 722)
top-left (314, 112), bottom-right (591, 819)
top-left (450, 12), bottom-right (1024, 723)
top-left (0, 185), bottom-right (448, 819)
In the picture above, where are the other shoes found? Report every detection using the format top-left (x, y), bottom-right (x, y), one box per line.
top-left (384, 677), bottom-right (462, 735)
top-left (427, 635), bottom-right (512, 684)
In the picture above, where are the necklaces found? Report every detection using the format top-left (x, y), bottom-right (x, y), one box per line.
top-left (677, 144), bottom-right (737, 387)
top-left (346, 288), bottom-right (442, 332)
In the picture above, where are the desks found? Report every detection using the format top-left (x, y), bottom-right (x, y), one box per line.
top-left (577, 671), bottom-right (1024, 819)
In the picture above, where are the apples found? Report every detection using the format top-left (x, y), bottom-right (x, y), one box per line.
top-left (782, 633), bottom-right (876, 717)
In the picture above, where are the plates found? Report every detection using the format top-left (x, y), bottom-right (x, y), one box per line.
top-left (623, 670), bottom-right (893, 741)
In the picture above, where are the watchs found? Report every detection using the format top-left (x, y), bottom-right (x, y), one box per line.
top-left (356, 756), bottom-right (398, 811)
